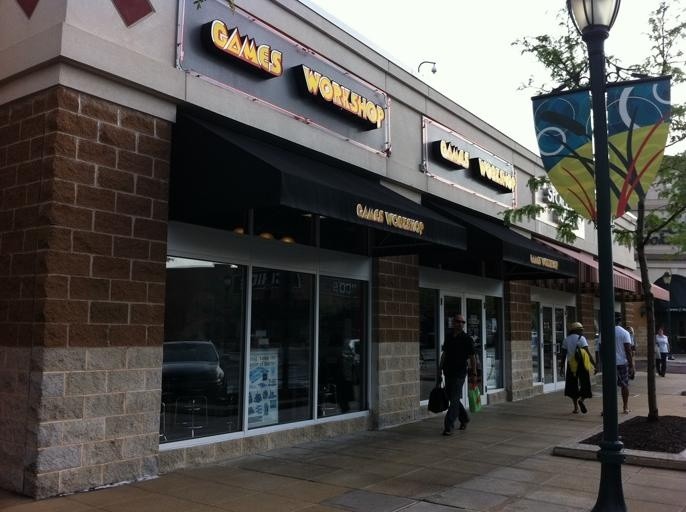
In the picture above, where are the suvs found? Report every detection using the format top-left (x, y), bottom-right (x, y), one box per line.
top-left (161, 339), bottom-right (230, 406)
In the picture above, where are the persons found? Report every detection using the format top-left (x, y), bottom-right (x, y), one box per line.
top-left (560, 322), bottom-right (598, 413)
top-left (595, 312), bottom-right (634, 416)
top-left (436, 315), bottom-right (477, 436)
top-left (664, 328), bottom-right (674, 360)
top-left (626, 327), bottom-right (637, 371)
top-left (656, 327), bottom-right (671, 377)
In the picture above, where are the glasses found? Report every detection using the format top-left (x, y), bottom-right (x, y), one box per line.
top-left (455, 319), bottom-right (465, 325)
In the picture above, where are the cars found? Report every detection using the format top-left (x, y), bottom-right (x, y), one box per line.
top-left (276, 365), bottom-right (311, 398)
top-left (342, 339), bottom-right (360, 385)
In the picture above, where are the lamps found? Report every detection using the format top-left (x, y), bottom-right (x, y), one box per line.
top-left (418, 61), bottom-right (436, 77)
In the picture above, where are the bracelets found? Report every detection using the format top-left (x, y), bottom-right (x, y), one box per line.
top-left (471, 373), bottom-right (476, 377)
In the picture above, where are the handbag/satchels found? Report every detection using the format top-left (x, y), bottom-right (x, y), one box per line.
top-left (568, 335), bottom-right (594, 378)
top-left (427, 379), bottom-right (450, 413)
top-left (468, 384), bottom-right (482, 413)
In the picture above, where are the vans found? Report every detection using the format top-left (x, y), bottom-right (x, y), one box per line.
top-left (530, 329), bottom-right (565, 366)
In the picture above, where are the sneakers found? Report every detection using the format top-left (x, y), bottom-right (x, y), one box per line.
top-left (443, 426), bottom-right (454, 436)
top-left (459, 417), bottom-right (470, 431)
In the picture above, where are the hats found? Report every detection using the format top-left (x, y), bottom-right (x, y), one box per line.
top-left (568, 321), bottom-right (584, 333)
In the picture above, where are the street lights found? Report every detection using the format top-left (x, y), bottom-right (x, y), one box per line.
top-left (661, 271), bottom-right (675, 361)
top-left (417, 60), bottom-right (437, 78)
top-left (557, 0), bottom-right (628, 512)
